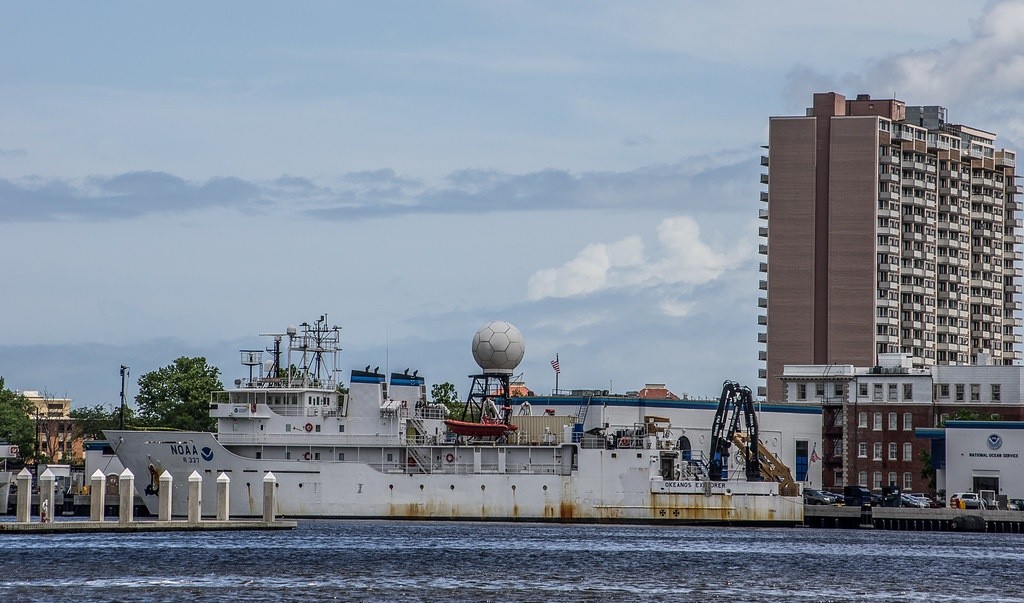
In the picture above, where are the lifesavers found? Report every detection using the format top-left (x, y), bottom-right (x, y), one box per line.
top-left (305, 422), bottom-right (313, 431)
top-left (445, 453), bottom-right (453, 462)
top-left (304, 451), bottom-right (312, 460)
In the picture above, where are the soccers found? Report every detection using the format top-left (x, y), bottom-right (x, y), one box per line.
top-left (471, 319), bottom-right (526, 371)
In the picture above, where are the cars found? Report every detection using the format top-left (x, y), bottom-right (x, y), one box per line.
top-left (1009, 501), bottom-right (1020, 512)
top-left (803, 484), bottom-right (944, 509)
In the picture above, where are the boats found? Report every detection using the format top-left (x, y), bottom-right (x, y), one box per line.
top-left (443, 419), bottom-right (519, 437)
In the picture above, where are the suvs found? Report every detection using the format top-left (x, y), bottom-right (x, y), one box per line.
top-left (950, 492), bottom-right (988, 510)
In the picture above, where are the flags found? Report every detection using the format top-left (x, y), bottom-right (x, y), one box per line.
top-left (550, 359), bottom-right (561, 374)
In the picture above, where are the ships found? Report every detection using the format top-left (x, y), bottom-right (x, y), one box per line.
top-left (102, 310), bottom-right (805, 526)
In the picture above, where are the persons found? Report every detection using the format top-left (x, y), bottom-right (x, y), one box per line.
top-left (41, 501), bottom-right (46, 524)
